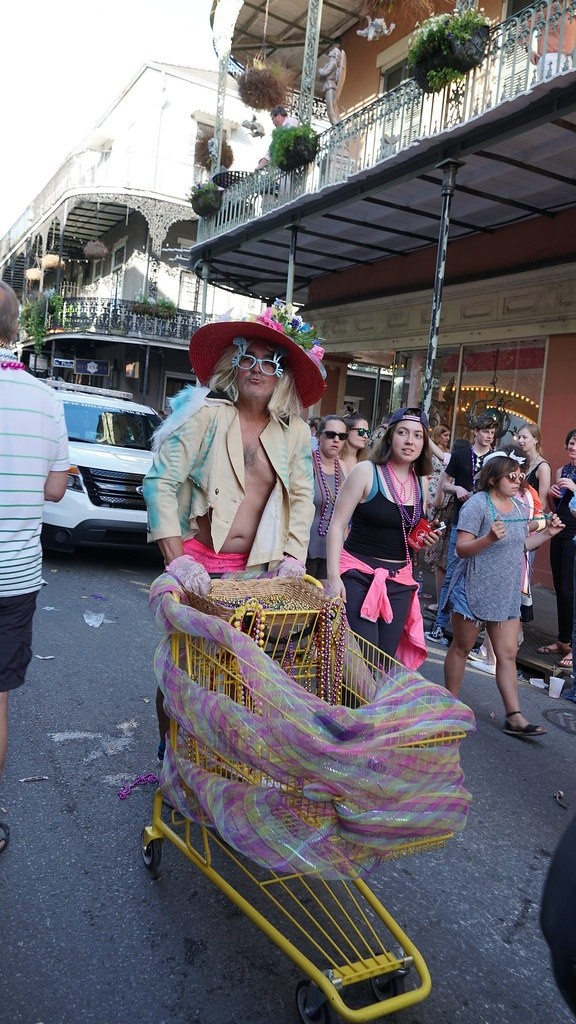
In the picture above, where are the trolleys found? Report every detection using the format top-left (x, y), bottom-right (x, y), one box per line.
top-left (141, 575), bottom-right (477, 1024)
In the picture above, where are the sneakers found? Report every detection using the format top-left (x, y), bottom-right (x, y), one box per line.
top-left (468, 648), bottom-right (486, 661)
top-left (426, 629), bottom-right (444, 642)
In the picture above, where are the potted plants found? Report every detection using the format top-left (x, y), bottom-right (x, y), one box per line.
top-left (186, 180), bottom-right (220, 214)
top-left (270, 124), bottom-right (318, 170)
top-left (19, 291), bottom-right (65, 357)
top-left (136, 293), bottom-right (176, 317)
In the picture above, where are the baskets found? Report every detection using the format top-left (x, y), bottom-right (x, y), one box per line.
top-left (182, 576), bottom-right (334, 627)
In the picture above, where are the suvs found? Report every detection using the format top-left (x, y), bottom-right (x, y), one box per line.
top-left (37, 378), bottom-right (164, 554)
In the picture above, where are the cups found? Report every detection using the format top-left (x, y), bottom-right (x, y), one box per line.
top-left (558, 486), bottom-right (567, 498)
top-left (406, 518), bottom-right (438, 553)
top-left (549, 676), bottom-right (565, 698)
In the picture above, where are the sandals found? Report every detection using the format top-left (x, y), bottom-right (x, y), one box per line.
top-left (537, 641), bottom-right (571, 653)
top-left (559, 657), bottom-right (573, 667)
top-left (504, 711), bottom-right (547, 735)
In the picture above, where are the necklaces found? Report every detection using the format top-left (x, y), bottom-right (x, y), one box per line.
top-left (0, 341), bottom-right (26, 371)
top-left (380, 459), bottom-right (421, 574)
top-left (486, 491), bottom-right (551, 523)
top-left (313, 450), bottom-right (341, 537)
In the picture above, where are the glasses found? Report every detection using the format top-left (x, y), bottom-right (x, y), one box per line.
top-left (232, 338), bottom-right (285, 378)
top-left (503, 472), bottom-right (525, 483)
top-left (320, 431), bottom-right (348, 440)
top-left (350, 428), bottom-right (371, 438)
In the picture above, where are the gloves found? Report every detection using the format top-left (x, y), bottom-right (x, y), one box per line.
top-left (167, 555), bottom-right (212, 596)
top-left (275, 556), bottom-right (306, 577)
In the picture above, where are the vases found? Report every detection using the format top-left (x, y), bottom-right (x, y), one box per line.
top-left (412, 24), bottom-right (488, 94)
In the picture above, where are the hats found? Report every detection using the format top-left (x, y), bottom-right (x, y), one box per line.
top-left (388, 407), bottom-right (429, 432)
top-left (189, 298), bottom-right (326, 408)
top-left (473, 450), bottom-right (526, 479)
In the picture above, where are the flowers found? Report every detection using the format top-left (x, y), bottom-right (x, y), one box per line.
top-left (407, 8), bottom-right (489, 62)
top-left (248, 299), bottom-right (324, 360)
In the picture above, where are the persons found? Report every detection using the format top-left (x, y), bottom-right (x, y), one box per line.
top-left (366, 412), bottom-right (392, 456)
top-left (427, 415), bottom-right (498, 642)
top-left (253, 105), bottom-right (298, 174)
top-left (0, 278), bottom-right (71, 853)
top-left (142, 304), bottom-right (326, 781)
top-left (305, 415), bottom-right (348, 581)
top-left (498, 445), bottom-right (545, 532)
top-left (517, 423), bottom-right (550, 511)
top-left (307, 416), bottom-right (319, 453)
top-left (318, 47), bottom-right (341, 125)
top-left (427, 424), bottom-right (450, 530)
top-left (443, 456), bottom-right (565, 735)
top-left (537, 429), bottom-right (576, 702)
top-left (340, 404), bottom-right (372, 471)
top-left (325, 407), bottom-right (442, 709)
top-left (424, 436), bottom-right (473, 615)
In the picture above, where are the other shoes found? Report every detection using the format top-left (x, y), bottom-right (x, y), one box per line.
top-left (563, 688), bottom-right (576, 702)
top-left (424, 601), bottom-right (439, 616)
top-left (470, 661), bottom-right (496, 674)
